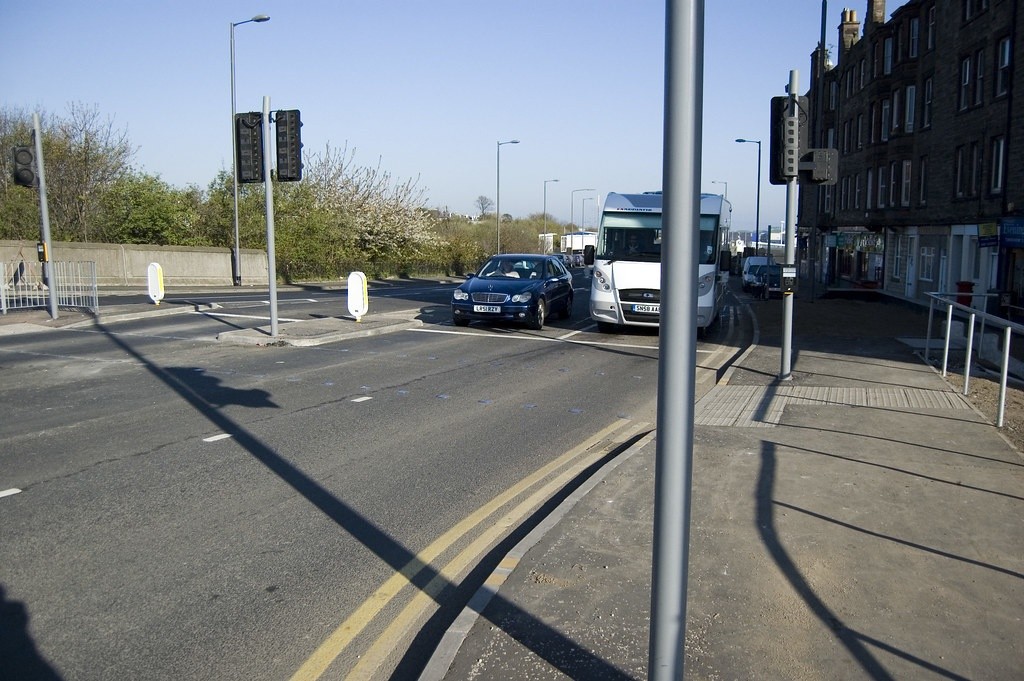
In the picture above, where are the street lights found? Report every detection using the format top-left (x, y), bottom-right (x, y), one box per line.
top-left (496, 138), bottom-right (521, 255)
top-left (232, 12), bottom-right (272, 285)
top-left (581, 197), bottom-right (595, 254)
top-left (734, 138), bottom-right (761, 256)
top-left (543, 179), bottom-right (560, 255)
top-left (571, 188), bottom-right (597, 255)
top-left (711, 181), bottom-right (727, 197)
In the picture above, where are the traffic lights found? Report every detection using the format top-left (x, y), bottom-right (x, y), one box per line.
top-left (13, 145), bottom-right (39, 188)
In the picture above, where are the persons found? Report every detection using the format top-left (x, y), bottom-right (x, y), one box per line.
top-left (486, 261), bottom-right (521, 279)
top-left (618, 234), bottom-right (647, 262)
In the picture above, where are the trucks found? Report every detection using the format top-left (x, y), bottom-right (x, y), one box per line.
top-left (582, 191), bottom-right (733, 336)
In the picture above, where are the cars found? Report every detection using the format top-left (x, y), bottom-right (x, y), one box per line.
top-left (550, 254), bottom-right (586, 269)
top-left (450, 254), bottom-right (575, 329)
top-left (738, 256), bottom-right (785, 298)
